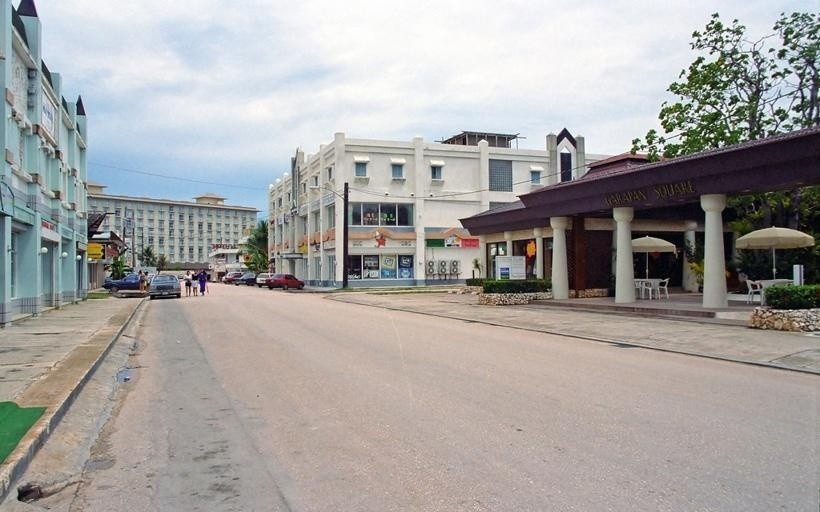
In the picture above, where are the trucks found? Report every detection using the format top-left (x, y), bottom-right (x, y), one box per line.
top-left (104, 266), bottom-right (156, 283)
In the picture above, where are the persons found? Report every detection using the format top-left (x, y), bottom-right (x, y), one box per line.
top-left (137, 268), bottom-right (208, 296)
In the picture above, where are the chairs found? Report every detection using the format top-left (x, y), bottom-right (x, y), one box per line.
top-left (635, 277), bottom-right (671, 300)
top-left (745, 278), bottom-right (794, 305)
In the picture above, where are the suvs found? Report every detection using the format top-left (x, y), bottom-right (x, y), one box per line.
top-left (226, 272), bottom-right (243, 283)
top-left (256, 273), bottom-right (273, 287)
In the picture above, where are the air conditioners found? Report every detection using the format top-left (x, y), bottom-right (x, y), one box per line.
top-left (426, 259), bottom-right (463, 275)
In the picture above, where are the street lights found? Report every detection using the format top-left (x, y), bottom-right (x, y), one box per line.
top-left (310, 182), bottom-right (349, 288)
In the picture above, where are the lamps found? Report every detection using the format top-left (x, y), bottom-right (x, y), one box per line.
top-left (38, 246), bottom-right (92, 263)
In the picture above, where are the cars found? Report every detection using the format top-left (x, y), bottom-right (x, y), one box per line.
top-left (148, 273), bottom-right (181, 299)
top-left (234, 273), bottom-right (257, 286)
top-left (266, 273), bottom-right (304, 290)
top-left (222, 276), bottom-right (228, 283)
top-left (103, 273), bottom-right (144, 292)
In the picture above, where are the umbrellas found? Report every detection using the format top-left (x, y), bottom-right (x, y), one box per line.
top-left (735, 226), bottom-right (815, 279)
top-left (631, 235), bottom-right (677, 279)
